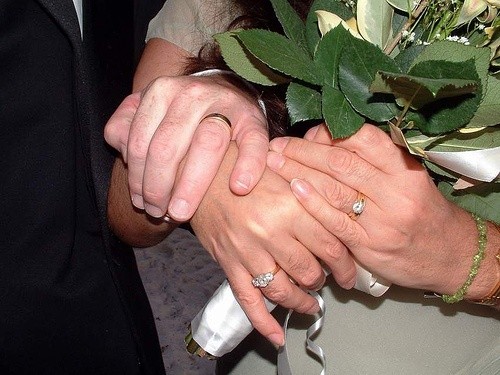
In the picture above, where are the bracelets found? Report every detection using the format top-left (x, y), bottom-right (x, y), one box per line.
top-left (431, 207), bottom-right (490, 304)
top-left (463, 217), bottom-right (500, 312)
top-left (187, 68), bottom-right (269, 124)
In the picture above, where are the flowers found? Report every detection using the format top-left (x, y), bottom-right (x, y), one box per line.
top-left (185, 1), bottom-right (500, 374)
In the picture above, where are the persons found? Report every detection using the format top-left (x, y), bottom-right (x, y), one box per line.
top-left (0, 0), bottom-right (273, 374)
top-left (102, 0), bottom-right (500, 375)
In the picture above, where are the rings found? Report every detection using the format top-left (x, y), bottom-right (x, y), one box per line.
top-left (199, 111), bottom-right (234, 143)
top-left (345, 189), bottom-right (368, 221)
top-left (250, 262), bottom-right (281, 288)
